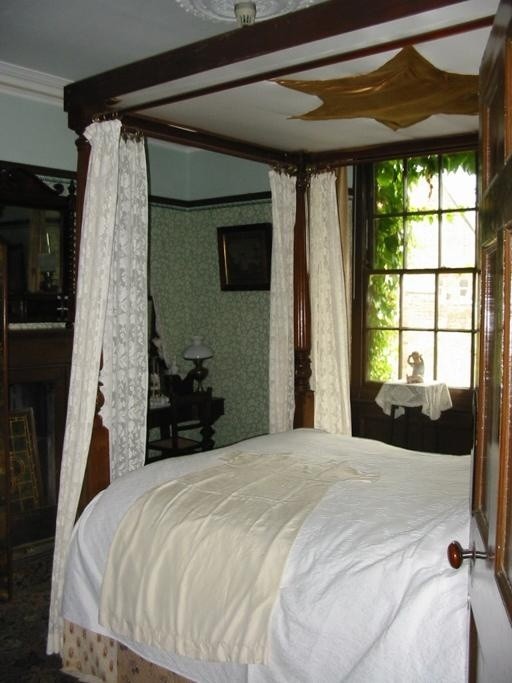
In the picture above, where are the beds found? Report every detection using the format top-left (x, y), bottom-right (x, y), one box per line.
top-left (43, 0), bottom-right (500, 683)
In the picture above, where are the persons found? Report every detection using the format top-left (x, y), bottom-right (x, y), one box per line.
top-left (406, 351), bottom-right (425, 384)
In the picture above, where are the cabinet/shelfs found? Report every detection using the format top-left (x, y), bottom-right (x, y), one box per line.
top-left (0, 172), bottom-right (81, 551)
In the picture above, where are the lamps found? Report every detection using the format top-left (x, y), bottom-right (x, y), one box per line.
top-left (183, 336), bottom-right (214, 392)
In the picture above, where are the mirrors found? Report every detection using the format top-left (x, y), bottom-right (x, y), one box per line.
top-left (2, 199), bottom-right (70, 330)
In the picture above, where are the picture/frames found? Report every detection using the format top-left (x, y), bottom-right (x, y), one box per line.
top-left (217, 224), bottom-right (272, 292)
